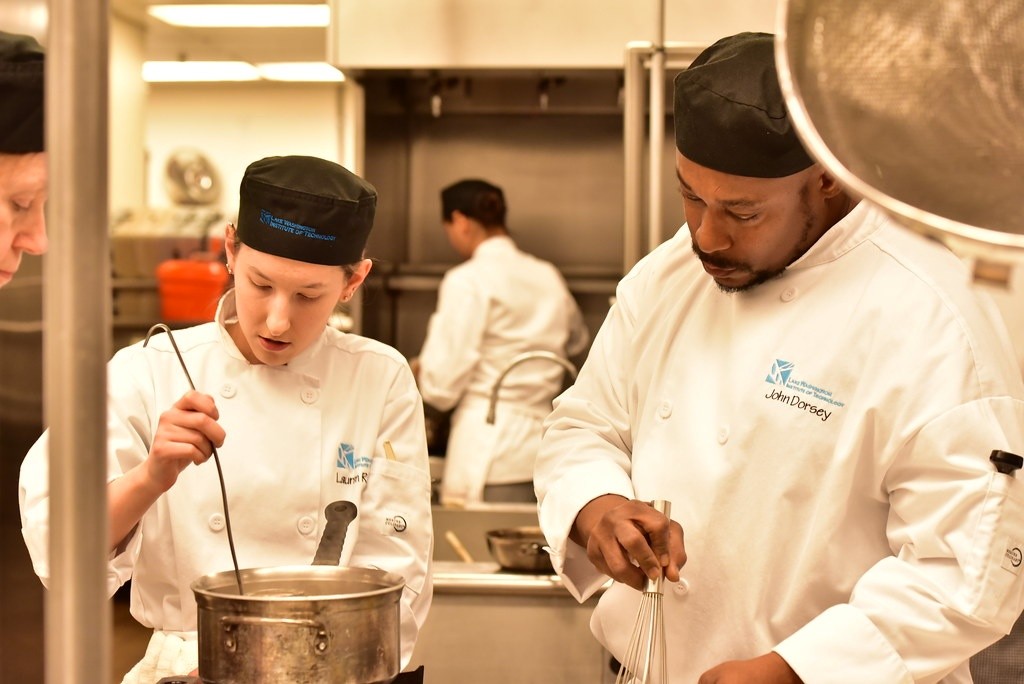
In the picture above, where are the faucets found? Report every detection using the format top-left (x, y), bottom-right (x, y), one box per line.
top-left (483, 349), bottom-right (577, 425)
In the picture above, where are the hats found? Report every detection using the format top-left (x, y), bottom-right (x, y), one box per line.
top-left (441, 178), bottom-right (504, 218)
top-left (673, 32), bottom-right (815, 178)
top-left (0, 29), bottom-right (46, 153)
top-left (236, 155), bottom-right (378, 266)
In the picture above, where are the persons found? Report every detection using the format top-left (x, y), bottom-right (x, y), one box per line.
top-left (409, 178), bottom-right (592, 507)
top-left (18, 155), bottom-right (433, 684)
top-left (0, 30), bottom-right (47, 292)
top-left (531, 32), bottom-right (1024, 684)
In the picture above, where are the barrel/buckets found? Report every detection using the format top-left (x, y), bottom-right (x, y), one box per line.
top-left (156, 259), bottom-right (226, 323)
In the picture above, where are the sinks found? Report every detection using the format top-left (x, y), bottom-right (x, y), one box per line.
top-left (423, 504), bottom-right (539, 565)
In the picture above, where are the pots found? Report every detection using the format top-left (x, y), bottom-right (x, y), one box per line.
top-left (486, 527), bottom-right (555, 573)
top-left (190, 500), bottom-right (405, 684)
